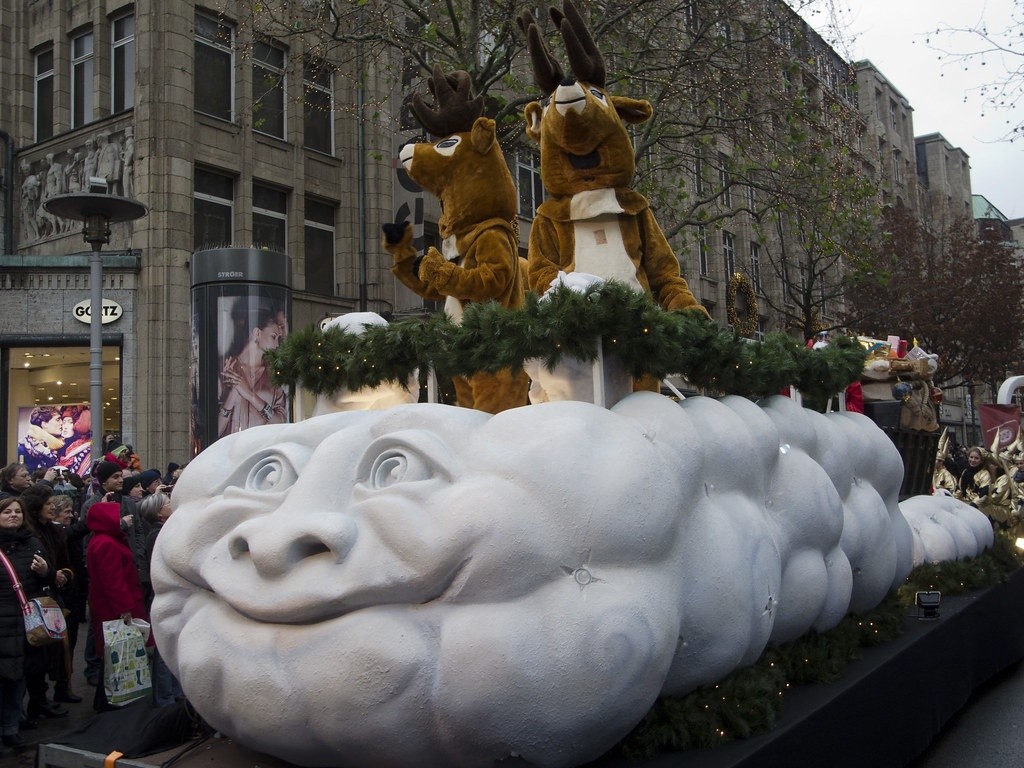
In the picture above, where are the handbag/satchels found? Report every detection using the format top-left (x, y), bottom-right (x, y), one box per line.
top-left (0, 546), bottom-right (67, 647)
top-left (102, 617), bottom-right (153, 707)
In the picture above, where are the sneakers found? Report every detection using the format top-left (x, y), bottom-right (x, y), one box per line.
top-left (53, 693), bottom-right (82, 702)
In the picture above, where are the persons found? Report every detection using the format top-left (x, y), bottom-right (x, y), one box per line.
top-left (213, 311), bottom-right (292, 442)
top-left (0, 404), bottom-right (187, 744)
top-left (935, 425), bottom-right (1023, 530)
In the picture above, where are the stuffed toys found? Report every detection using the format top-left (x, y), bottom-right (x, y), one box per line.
top-left (383, 60), bottom-right (530, 411)
top-left (512, 4), bottom-right (717, 410)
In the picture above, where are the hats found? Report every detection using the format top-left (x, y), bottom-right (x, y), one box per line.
top-left (139, 470), bottom-right (160, 489)
top-left (122, 477), bottom-right (142, 495)
top-left (168, 462), bottom-right (179, 475)
top-left (97, 461), bottom-right (122, 485)
top-left (60, 403), bottom-right (91, 421)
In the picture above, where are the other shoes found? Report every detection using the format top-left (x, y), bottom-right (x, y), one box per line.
top-left (19, 719), bottom-right (40, 729)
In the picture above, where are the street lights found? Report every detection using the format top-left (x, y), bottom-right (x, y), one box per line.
top-left (45, 177), bottom-right (148, 468)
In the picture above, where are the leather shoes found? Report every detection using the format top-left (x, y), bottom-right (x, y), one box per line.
top-left (2, 734), bottom-right (25, 746)
top-left (27, 702), bottom-right (69, 719)
